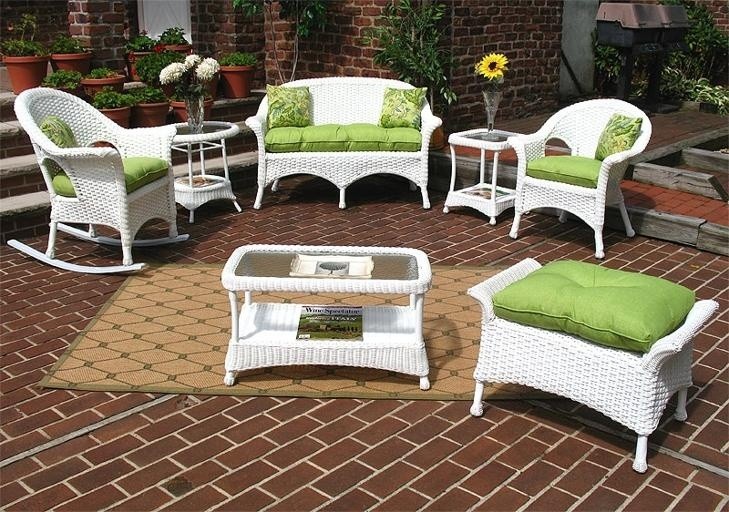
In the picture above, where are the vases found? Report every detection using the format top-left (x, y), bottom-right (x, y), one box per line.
top-left (481, 88), bottom-right (505, 142)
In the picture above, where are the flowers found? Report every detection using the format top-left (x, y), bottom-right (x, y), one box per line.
top-left (471, 52), bottom-right (510, 107)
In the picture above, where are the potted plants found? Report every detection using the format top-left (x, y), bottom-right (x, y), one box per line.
top-left (1, 10), bottom-right (260, 135)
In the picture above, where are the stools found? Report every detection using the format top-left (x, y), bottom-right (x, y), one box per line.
top-left (456, 254), bottom-right (720, 476)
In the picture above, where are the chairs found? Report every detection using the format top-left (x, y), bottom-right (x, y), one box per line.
top-left (4, 86), bottom-right (196, 277)
top-left (505, 95), bottom-right (654, 263)
top-left (241, 75), bottom-right (443, 213)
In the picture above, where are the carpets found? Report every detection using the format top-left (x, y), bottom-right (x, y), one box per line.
top-left (32, 261), bottom-right (566, 405)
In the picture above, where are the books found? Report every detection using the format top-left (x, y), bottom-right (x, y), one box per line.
top-left (295, 305), bottom-right (363, 342)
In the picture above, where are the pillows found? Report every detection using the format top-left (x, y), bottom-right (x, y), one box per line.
top-left (375, 85), bottom-right (429, 133)
top-left (593, 112), bottom-right (643, 163)
top-left (262, 80), bottom-right (313, 132)
top-left (35, 114), bottom-right (79, 180)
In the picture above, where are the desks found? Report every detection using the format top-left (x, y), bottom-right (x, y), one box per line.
top-left (157, 119), bottom-right (244, 224)
top-left (438, 128), bottom-right (532, 227)
